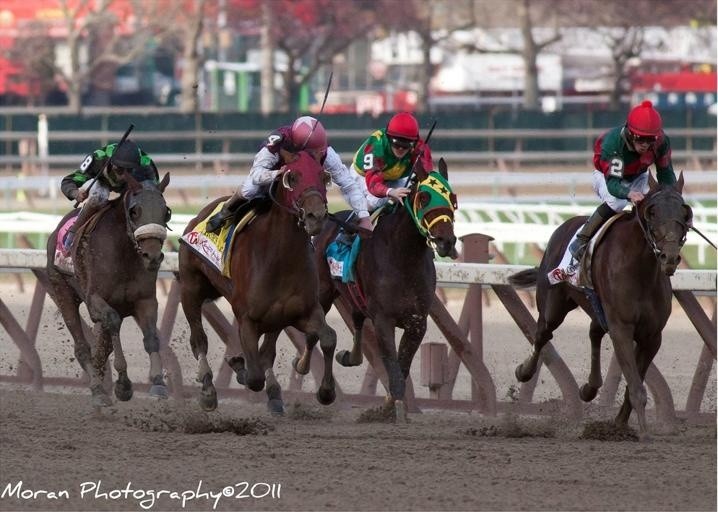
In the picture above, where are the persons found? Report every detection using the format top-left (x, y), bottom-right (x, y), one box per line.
top-left (206, 116), bottom-right (375, 239)
top-left (62, 139), bottom-right (160, 252)
top-left (569, 100), bottom-right (678, 263)
top-left (336, 110), bottom-right (435, 249)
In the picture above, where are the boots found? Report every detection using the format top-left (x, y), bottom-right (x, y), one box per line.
top-left (65, 203), bottom-right (96, 257)
top-left (336, 210), bottom-right (356, 249)
top-left (569, 209), bottom-right (605, 265)
top-left (206, 185), bottom-right (249, 233)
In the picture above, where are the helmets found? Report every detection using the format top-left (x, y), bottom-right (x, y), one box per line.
top-left (292, 116), bottom-right (327, 157)
top-left (112, 140), bottom-right (140, 169)
top-left (628, 101), bottom-right (662, 136)
top-left (387, 114), bottom-right (419, 140)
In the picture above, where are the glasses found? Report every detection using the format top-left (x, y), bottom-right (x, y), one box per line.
top-left (628, 130), bottom-right (658, 143)
top-left (390, 139), bottom-right (417, 149)
top-left (115, 166), bottom-right (133, 175)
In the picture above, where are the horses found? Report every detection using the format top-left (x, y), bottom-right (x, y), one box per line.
top-left (46, 170), bottom-right (170, 408)
top-left (178, 144), bottom-right (337, 413)
top-left (506, 168), bottom-right (688, 433)
top-left (291, 156), bottom-right (457, 428)
